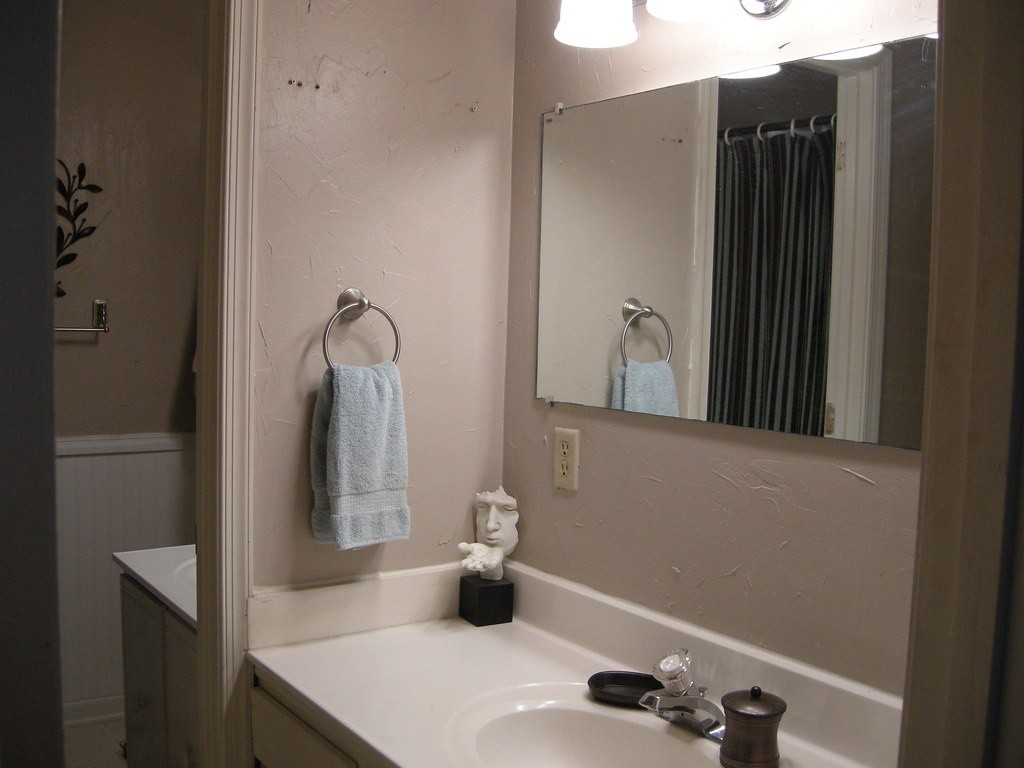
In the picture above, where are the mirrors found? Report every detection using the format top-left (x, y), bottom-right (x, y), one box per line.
top-left (532, 31), bottom-right (939, 452)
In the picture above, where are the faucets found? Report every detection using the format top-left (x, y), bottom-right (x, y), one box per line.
top-left (638, 654), bottom-right (728, 745)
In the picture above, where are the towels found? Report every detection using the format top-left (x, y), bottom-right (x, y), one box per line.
top-left (308, 360), bottom-right (413, 552)
top-left (610, 358), bottom-right (681, 422)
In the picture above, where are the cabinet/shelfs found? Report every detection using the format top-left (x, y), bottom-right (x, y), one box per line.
top-left (118, 573), bottom-right (195, 767)
top-left (249, 678), bottom-right (359, 768)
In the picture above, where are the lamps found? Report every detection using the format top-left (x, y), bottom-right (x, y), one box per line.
top-left (554, 0), bottom-right (792, 50)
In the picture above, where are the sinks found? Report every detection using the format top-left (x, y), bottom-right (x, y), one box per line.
top-left (444, 682), bottom-right (726, 767)
